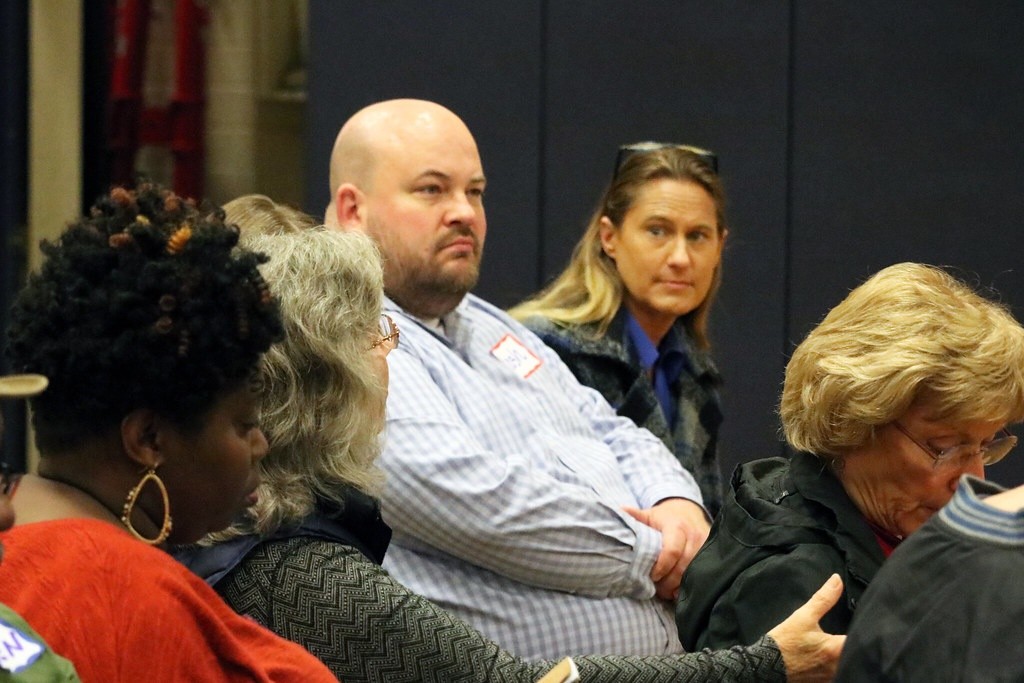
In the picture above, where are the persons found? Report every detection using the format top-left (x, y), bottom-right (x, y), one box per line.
top-left (203, 227), bottom-right (851, 683)
top-left (320, 97), bottom-right (712, 663)
top-left (218, 193), bottom-right (318, 246)
top-left (837, 471), bottom-right (1024, 683)
top-left (508, 140), bottom-right (731, 519)
top-left (0, 183), bottom-right (335, 683)
top-left (677, 261), bottom-right (1024, 683)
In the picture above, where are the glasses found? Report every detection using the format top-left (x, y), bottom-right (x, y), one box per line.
top-left (0, 462), bottom-right (22, 503)
top-left (611, 141), bottom-right (719, 183)
top-left (360, 315), bottom-right (399, 357)
top-left (892, 419), bottom-right (1019, 474)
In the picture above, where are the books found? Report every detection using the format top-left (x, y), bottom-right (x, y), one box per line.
top-left (537, 656), bottom-right (580, 683)
